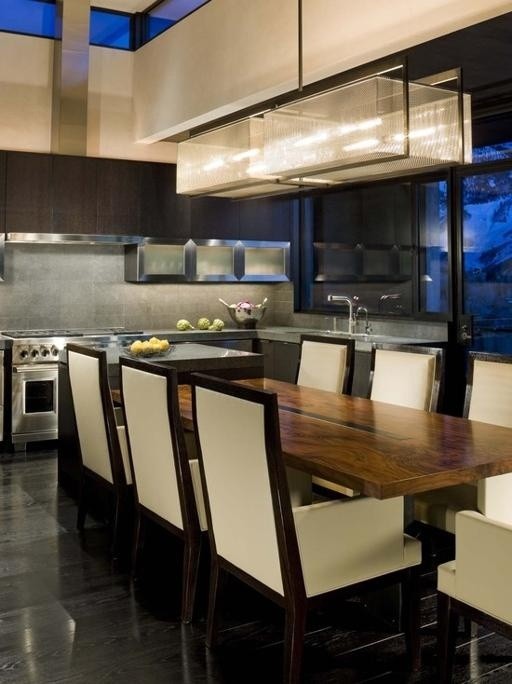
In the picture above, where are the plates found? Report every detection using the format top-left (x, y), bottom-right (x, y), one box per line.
top-left (124, 345), bottom-right (175, 358)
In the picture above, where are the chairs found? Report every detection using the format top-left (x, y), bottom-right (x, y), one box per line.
top-left (311, 340), bottom-right (446, 502)
top-left (68, 343), bottom-right (139, 583)
top-left (436, 508), bottom-right (511, 682)
top-left (118, 354), bottom-right (209, 625)
top-left (294, 335), bottom-right (356, 398)
top-left (414, 350), bottom-right (511, 536)
top-left (190, 371), bottom-right (424, 682)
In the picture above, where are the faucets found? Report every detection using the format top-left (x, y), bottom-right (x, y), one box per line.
top-left (357, 306), bottom-right (370, 336)
top-left (328, 293), bottom-right (357, 333)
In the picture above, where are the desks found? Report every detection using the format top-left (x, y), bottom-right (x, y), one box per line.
top-left (110, 376), bottom-right (510, 502)
top-left (97, 342), bottom-right (264, 390)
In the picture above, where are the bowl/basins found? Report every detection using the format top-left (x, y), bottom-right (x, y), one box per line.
top-left (228, 307), bottom-right (266, 329)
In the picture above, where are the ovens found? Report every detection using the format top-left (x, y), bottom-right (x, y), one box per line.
top-left (12, 345), bottom-right (63, 444)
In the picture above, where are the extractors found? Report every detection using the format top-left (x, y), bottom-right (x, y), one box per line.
top-left (0, 1), bottom-right (176, 246)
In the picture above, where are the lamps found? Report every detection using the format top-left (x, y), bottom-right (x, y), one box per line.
top-left (176, 76), bottom-right (472, 201)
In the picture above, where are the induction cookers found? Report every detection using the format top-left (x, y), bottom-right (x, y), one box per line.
top-left (2, 328), bottom-right (142, 337)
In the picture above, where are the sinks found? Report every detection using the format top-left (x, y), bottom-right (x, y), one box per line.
top-left (286, 331), bottom-right (366, 339)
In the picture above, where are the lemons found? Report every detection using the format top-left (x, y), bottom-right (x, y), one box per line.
top-left (131, 337), bottom-right (170, 353)
